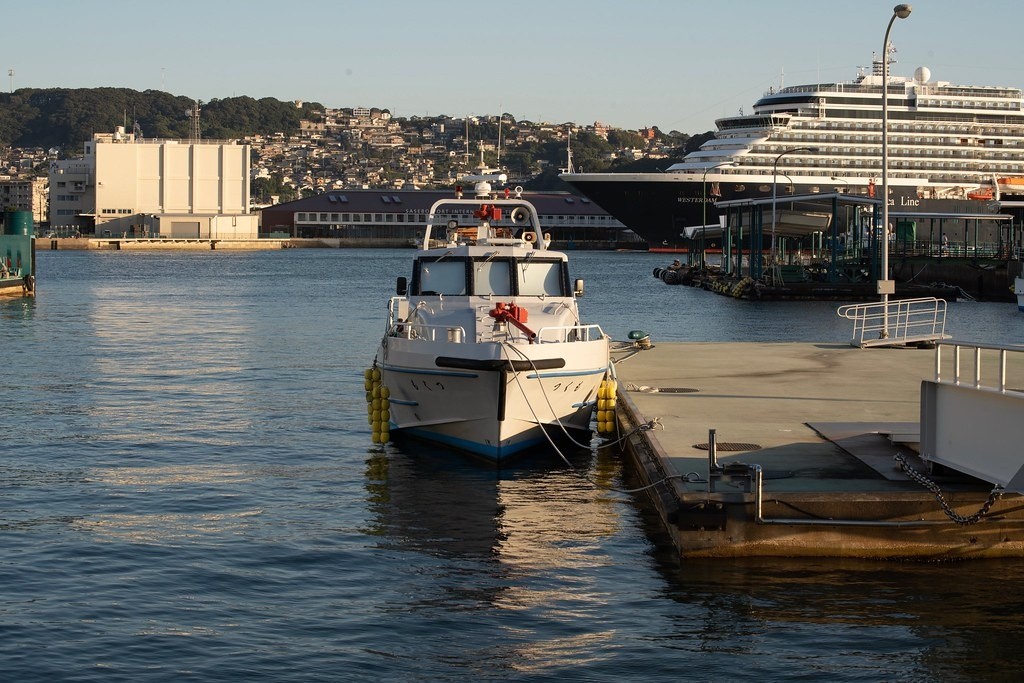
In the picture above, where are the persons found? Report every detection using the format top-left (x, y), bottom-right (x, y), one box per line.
top-left (942, 233), bottom-right (947, 245)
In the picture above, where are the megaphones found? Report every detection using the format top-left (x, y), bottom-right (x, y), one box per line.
top-left (511, 207), bottom-right (529, 224)
top-left (522, 232), bottom-right (537, 243)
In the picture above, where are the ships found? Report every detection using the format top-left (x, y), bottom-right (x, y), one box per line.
top-left (558, 41), bottom-right (1024, 254)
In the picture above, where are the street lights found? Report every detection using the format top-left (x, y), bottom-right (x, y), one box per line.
top-left (701, 162), bottom-right (741, 265)
top-left (770, 145), bottom-right (821, 277)
top-left (879, 2), bottom-right (915, 340)
top-left (770, 170), bottom-right (794, 264)
top-left (830, 176), bottom-right (849, 256)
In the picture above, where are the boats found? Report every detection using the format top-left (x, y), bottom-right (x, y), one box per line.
top-left (998, 178), bottom-right (1024, 190)
top-left (374, 105), bottom-right (613, 464)
top-left (967, 188), bottom-right (993, 200)
